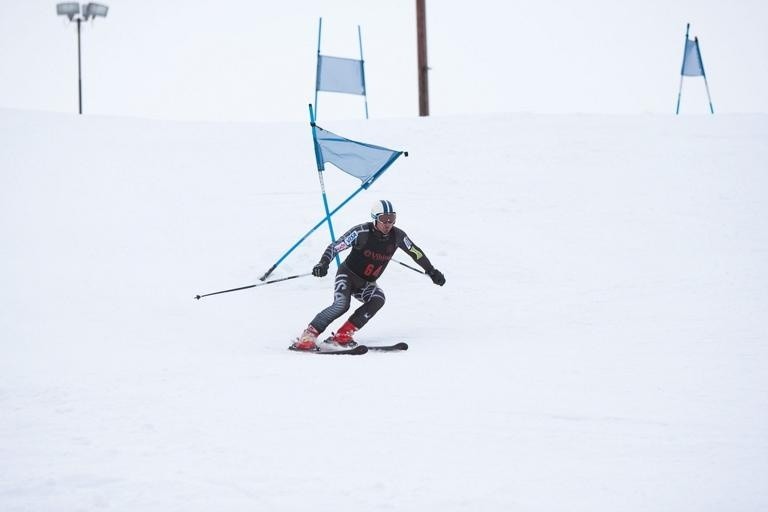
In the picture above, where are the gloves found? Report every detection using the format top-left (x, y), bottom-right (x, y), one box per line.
top-left (425, 265), bottom-right (445, 286)
top-left (313, 257), bottom-right (330, 277)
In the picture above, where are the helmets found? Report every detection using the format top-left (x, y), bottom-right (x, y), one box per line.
top-left (371, 200), bottom-right (393, 230)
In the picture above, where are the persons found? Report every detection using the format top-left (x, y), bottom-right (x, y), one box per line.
top-left (294, 199), bottom-right (444, 350)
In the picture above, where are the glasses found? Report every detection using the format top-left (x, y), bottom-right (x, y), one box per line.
top-left (371, 213), bottom-right (396, 224)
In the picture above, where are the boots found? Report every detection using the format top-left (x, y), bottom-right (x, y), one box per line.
top-left (295, 325), bottom-right (320, 350)
top-left (333, 320), bottom-right (358, 347)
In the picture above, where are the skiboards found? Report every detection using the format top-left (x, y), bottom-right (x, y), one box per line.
top-left (289, 340), bottom-right (409, 355)
